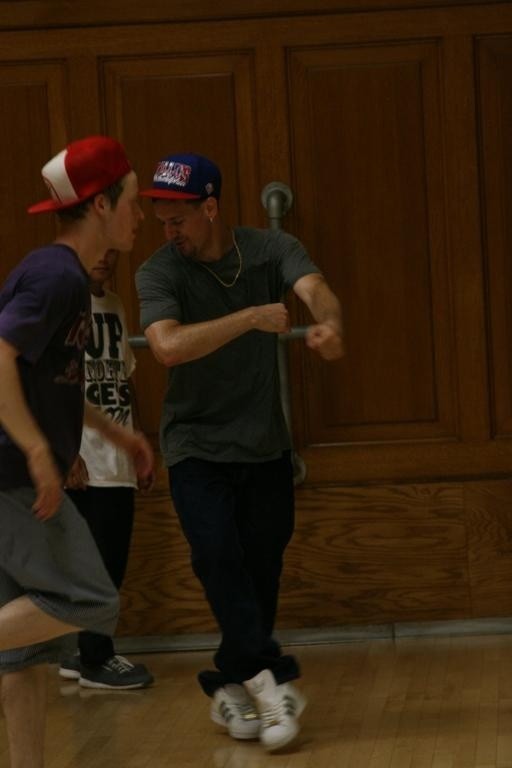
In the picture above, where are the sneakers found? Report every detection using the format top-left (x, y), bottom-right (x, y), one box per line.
top-left (209, 678), bottom-right (262, 740)
top-left (57, 649), bottom-right (82, 680)
top-left (79, 654), bottom-right (155, 690)
top-left (241, 667), bottom-right (309, 753)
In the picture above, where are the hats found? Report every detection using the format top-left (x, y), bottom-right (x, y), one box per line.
top-left (137, 152), bottom-right (223, 203)
top-left (26, 135), bottom-right (132, 215)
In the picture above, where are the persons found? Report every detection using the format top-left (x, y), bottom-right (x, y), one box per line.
top-left (134, 150), bottom-right (346, 753)
top-left (59, 247), bottom-right (157, 695)
top-left (0, 134), bottom-right (158, 653)
